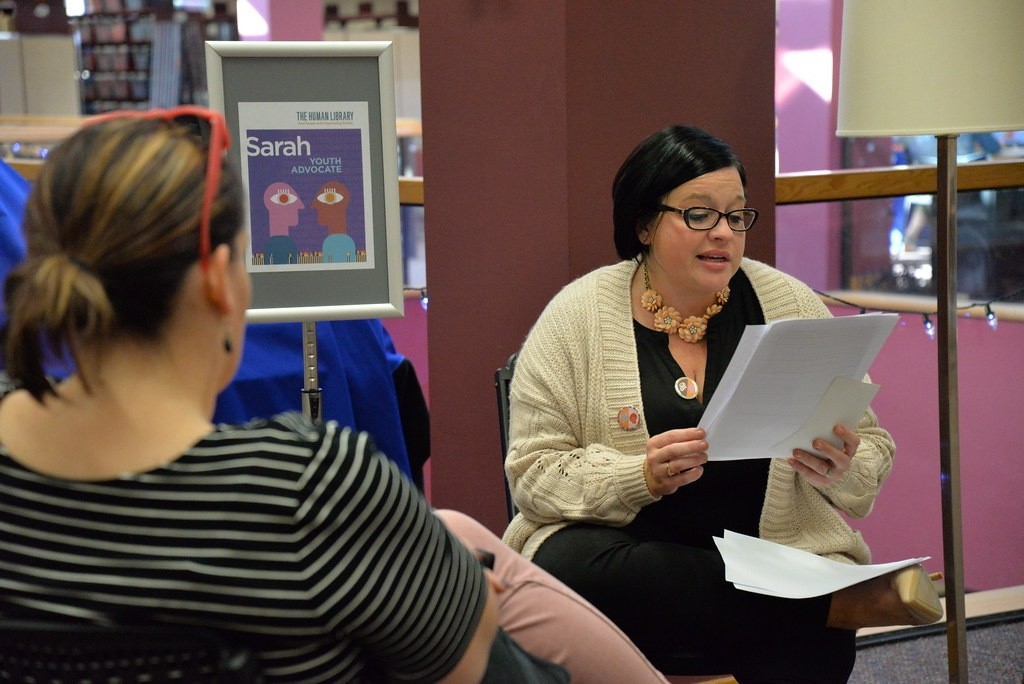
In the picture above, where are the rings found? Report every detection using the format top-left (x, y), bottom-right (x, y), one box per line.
top-left (824, 463), bottom-right (834, 478)
top-left (666, 460), bottom-right (675, 477)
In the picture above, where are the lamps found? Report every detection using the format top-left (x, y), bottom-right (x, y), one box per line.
top-left (834, 2), bottom-right (1023, 684)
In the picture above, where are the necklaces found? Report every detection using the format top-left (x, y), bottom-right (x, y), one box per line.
top-left (641, 256), bottom-right (730, 344)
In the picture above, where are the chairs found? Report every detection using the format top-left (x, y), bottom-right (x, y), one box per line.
top-left (496, 349), bottom-right (736, 683)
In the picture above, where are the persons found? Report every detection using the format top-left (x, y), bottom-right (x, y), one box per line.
top-left (0, 105), bottom-right (669, 684)
top-left (498, 123), bottom-right (943, 684)
top-left (903, 133), bottom-right (1002, 250)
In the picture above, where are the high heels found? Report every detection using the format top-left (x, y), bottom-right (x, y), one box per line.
top-left (894, 563), bottom-right (946, 627)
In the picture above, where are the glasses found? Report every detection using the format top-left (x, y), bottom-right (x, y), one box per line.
top-left (82, 106), bottom-right (226, 314)
top-left (654, 204), bottom-right (759, 232)
